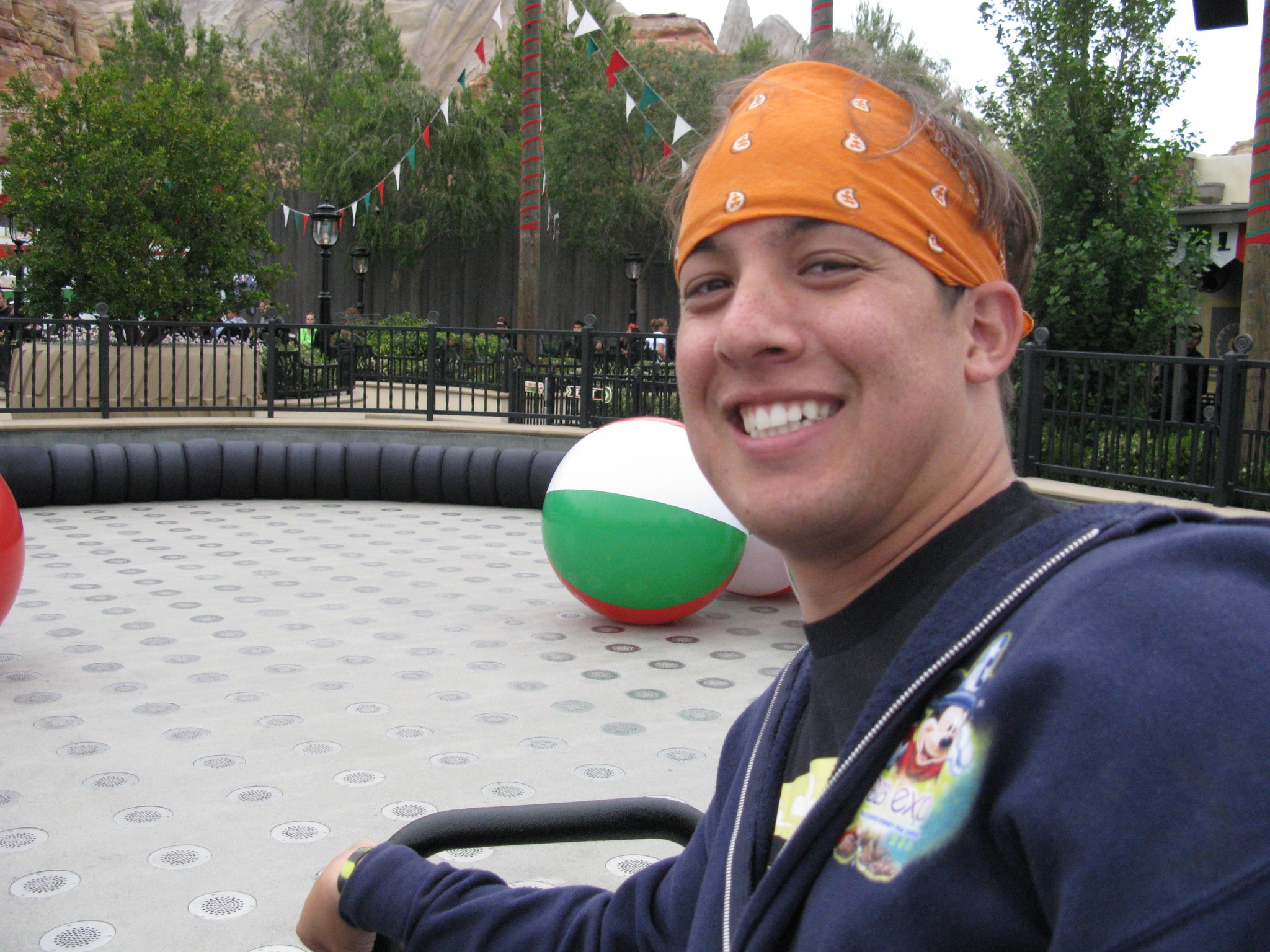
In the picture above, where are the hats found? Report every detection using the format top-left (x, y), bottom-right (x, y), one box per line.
top-left (628, 323), bottom-right (639, 329)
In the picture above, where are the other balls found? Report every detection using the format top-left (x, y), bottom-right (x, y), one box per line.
top-left (0, 473), bottom-right (27, 626)
top-left (723, 533), bottom-right (792, 600)
top-left (540, 415), bottom-right (748, 627)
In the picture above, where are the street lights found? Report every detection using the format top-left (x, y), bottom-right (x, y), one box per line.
top-left (350, 246), bottom-right (370, 318)
top-left (8, 213), bottom-right (34, 317)
top-left (623, 251), bottom-right (646, 367)
top-left (310, 197), bottom-right (342, 344)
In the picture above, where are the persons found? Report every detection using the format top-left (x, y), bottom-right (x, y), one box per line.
top-left (1162, 324), bottom-right (1208, 422)
top-left (293, 42), bottom-right (1269, 952)
top-left (0, 276), bottom-right (675, 406)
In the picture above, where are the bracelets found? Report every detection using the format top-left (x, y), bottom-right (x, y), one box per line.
top-left (334, 845), bottom-right (374, 895)
